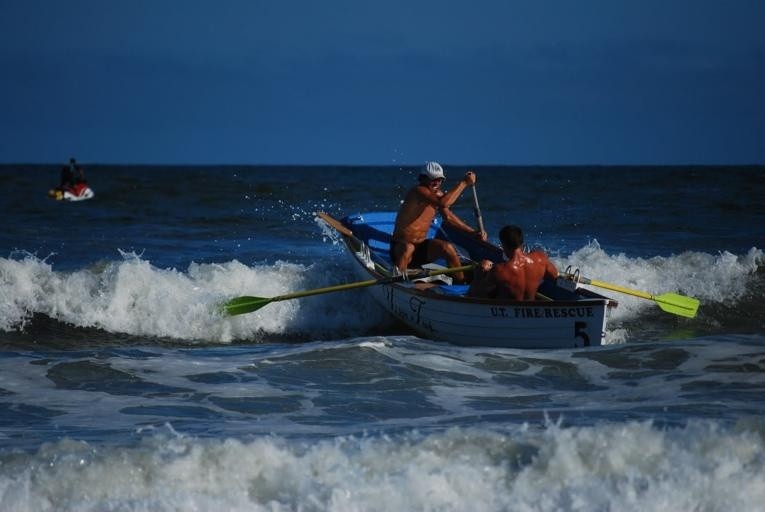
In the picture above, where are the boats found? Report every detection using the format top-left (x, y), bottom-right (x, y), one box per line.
top-left (340, 211), bottom-right (619, 349)
top-left (48, 182), bottom-right (95, 201)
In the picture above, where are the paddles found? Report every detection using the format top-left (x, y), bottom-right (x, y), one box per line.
top-left (319, 213), bottom-right (391, 269)
top-left (559, 273), bottom-right (700, 319)
top-left (226, 265), bottom-right (479, 316)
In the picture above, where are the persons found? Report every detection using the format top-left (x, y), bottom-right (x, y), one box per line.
top-left (59, 158), bottom-right (87, 190)
top-left (469, 225), bottom-right (558, 301)
top-left (389, 161), bottom-right (477, 284)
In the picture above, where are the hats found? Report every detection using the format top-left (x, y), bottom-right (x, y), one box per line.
top-left (419, 161), bottom-right (447, 181)
